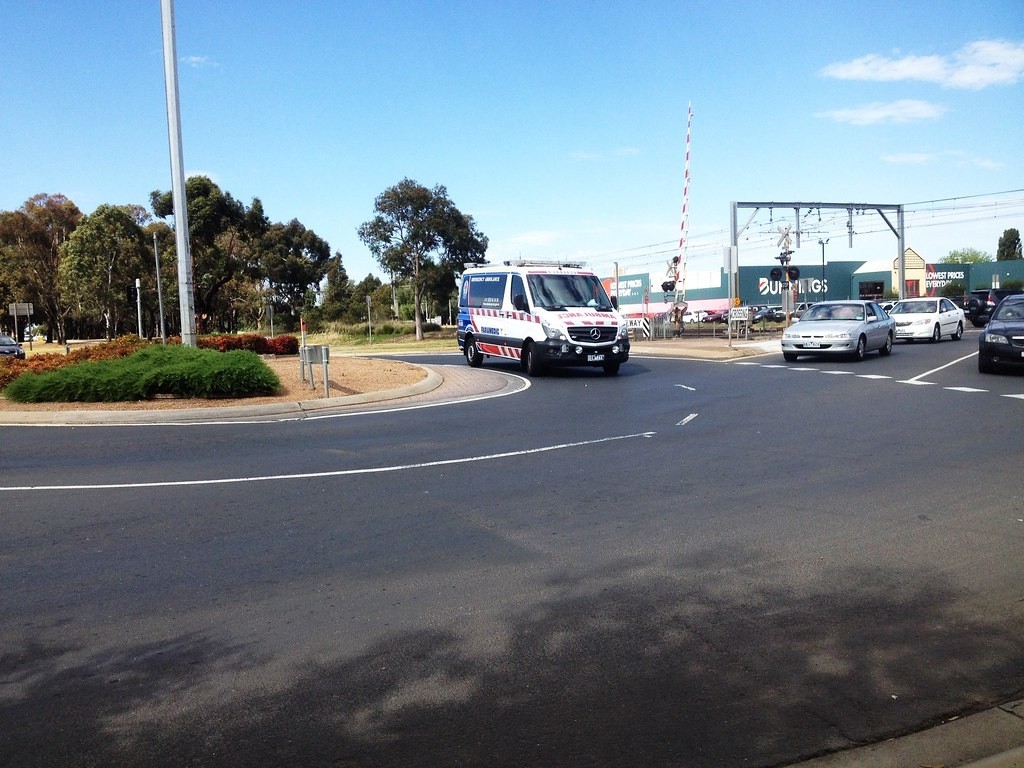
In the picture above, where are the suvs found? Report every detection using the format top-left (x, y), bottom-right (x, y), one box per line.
top-left (978, 294), bottom-right (1024, 375)
top-left (964, 289), bottom-right (1024, 328)
top-left (748, 305), bottom-right (768, 314)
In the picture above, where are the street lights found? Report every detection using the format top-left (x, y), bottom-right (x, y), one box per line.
top-left (819, 239), bottom-right (830, 302)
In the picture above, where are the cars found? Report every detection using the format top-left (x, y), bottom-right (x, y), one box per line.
top-left (781, 300), bottom-right (896, 362)
top-left (702, 309), bottom-right (728, 324)
top-left (858, 301), bottom-right (899, 319)
top-left (671, 309), bottom-right (708, 324)
top-left (888, 296), bottom-right (965, 343)
top-left (0, 334), bottom-right (26, 360)
top-left (721, 312), bottom-right (728, 324)
top-left (752, 306), bottom-right (782, 323)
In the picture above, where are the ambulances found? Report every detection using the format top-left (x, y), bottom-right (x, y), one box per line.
top-left (457, 256), bottom-right (631, 375)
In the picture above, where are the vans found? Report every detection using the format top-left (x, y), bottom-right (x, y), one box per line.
top-left (773, 302), bottom-right (816, 323)
